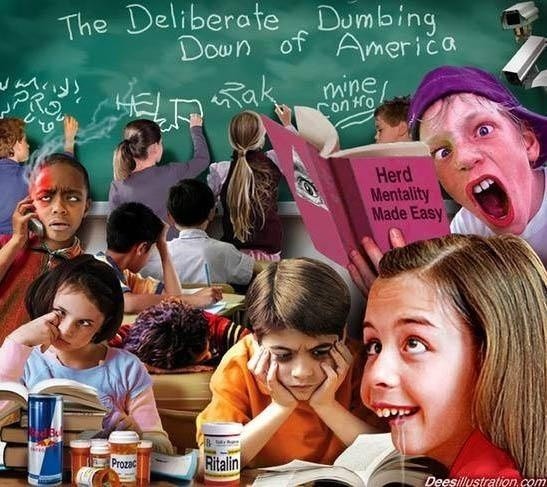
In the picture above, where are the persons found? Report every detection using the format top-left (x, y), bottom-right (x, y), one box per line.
top-left (0, 66), bottom-right (547, 487)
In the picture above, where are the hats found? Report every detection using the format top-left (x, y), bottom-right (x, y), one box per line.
top-left (407, 66), bottom-right (546, 169)
